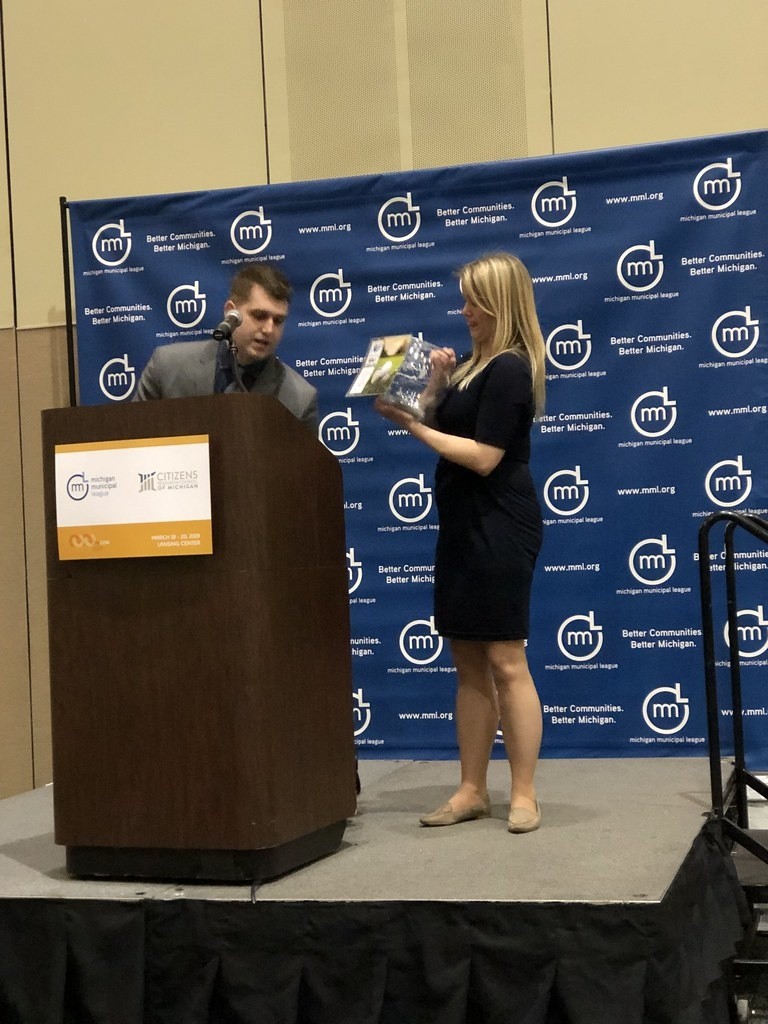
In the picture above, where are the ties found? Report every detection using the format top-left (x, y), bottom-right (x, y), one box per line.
top-left (223, 364), bottom-right (246, 395)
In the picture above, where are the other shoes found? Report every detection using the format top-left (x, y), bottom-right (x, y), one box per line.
top-left (506, 799), bottom-right (542, 833)
top-left (356, 773), bottom-right (361, 795)
top-left (419, 793), bottom-right (492, 826)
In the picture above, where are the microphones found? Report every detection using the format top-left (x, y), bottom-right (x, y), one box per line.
top-left (213, 310), bottom-right (243, 341)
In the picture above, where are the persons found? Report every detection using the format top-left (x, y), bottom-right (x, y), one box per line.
top-left (375, 248), bottom-right (547, 832)
top-left (368, 340), bottom-right (433, 409)
top-left (131, 264), bottom-right (320, 444)
